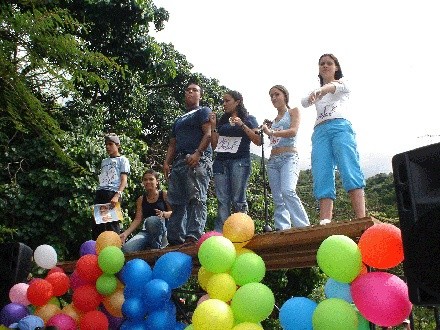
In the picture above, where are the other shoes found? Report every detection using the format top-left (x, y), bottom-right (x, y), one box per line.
top-left (185, 237), bottom-right (195, 242)
top-left (166, 243), bottom-right (172, 247)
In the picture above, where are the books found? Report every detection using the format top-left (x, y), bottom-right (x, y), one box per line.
top-left (91, 202), bottom-right (123, 224)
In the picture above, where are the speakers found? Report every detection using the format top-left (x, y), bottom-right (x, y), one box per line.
top-left (0, 241), bottom-right (33, 290)
top-left (392, 142), bottom-right (440, 307)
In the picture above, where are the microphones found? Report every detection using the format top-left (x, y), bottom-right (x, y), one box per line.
top-left (186, 154), bottom-right (190, 159)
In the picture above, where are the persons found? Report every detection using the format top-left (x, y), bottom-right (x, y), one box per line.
top-left (302, 54), bottom-right (366, 226)
top-left (163, 84), bottom-right (211, 246)
top-left (118, 169), bottom-right (174, 253)
top-left (91, 133), bottom-right (131, 241)
top-left (262, 85), bottom-right (311, 230)
top-left (208, 90), bottom-right (264, 236)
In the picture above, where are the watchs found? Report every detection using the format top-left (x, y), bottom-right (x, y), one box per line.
top-left (195, 149), bottom-right (205, 156)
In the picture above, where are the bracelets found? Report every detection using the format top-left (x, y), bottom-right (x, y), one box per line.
top-left (210, 129), bottom-right (217, 134)
top-left (115, 191), bottom-right (124, 197)
top-left (240, 123), bottom-right (245, 128)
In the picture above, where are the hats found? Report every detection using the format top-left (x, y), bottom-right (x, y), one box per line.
top-left (105, 133), bottom-right (121, 151)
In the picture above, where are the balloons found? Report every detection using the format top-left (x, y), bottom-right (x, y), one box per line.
top-left (0, 212), bottom-right (413, 330)
